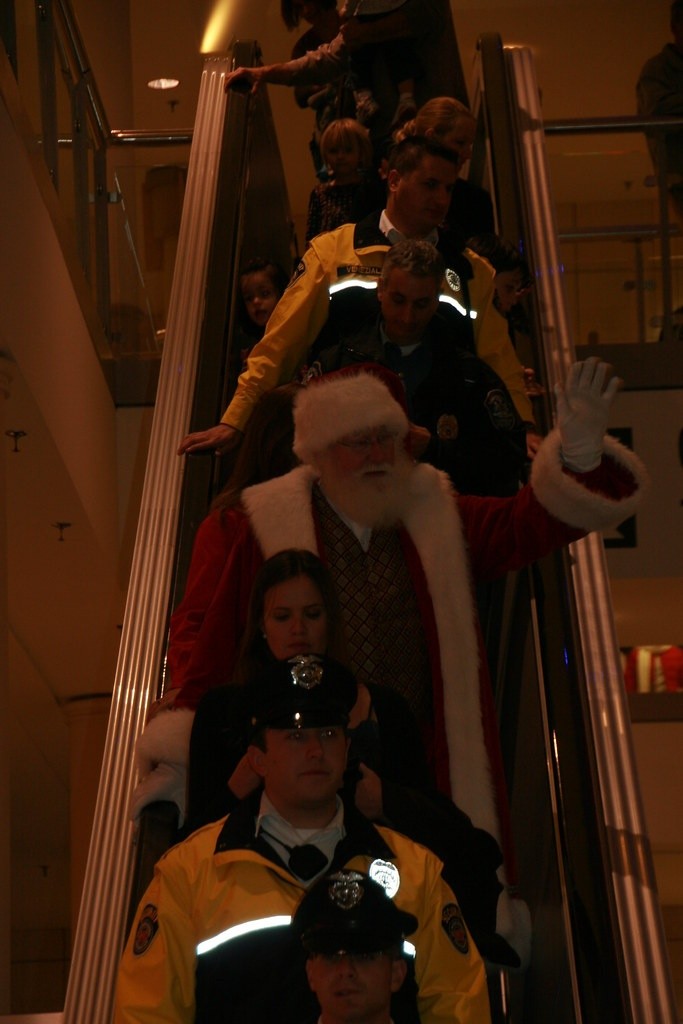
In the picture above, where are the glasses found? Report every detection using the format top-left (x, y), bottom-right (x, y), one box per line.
top-left (308, 947), bottom-right (385, 966)
top-left (337, 434), bottom-right (393, 454)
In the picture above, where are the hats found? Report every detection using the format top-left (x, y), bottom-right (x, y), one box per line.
top-left (250, 653), bottom-right (358, 730)
top-left (292, 362), bottom-right (409, 459)
top-left (275, 869), bottom-right (418, 974)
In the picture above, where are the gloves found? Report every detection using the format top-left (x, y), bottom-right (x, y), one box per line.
top-left (129, 761), bottom-right (187, 828)
top-left (554, 357), bottom-right (623, 473)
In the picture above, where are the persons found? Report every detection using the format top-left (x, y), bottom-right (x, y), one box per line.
top-left (121, 0), bottom-right (647, 1021)
top-left (634, 0), bottom-right (683, 339)
